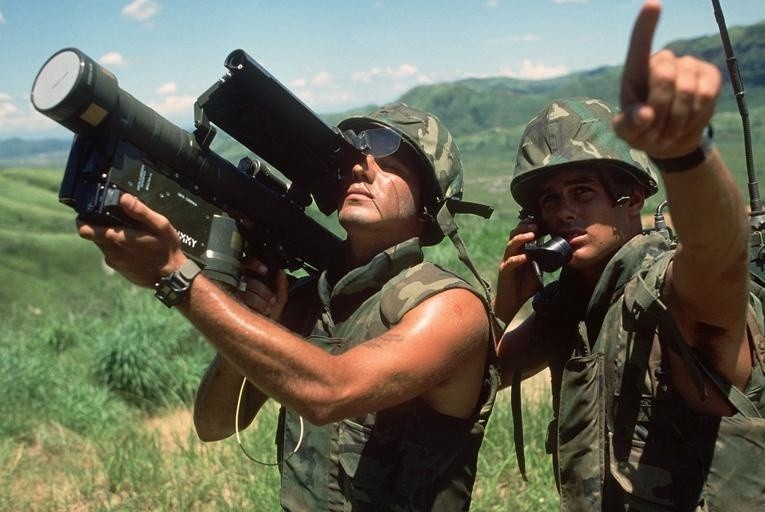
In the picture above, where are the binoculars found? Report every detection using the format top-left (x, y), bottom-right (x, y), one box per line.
top-left (30, 47), bottom-right (348, 292)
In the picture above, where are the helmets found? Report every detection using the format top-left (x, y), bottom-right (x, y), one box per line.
top-left (511, 97), bottom-right (658, 215)
top-left (338, 102), bottom-right (464, 244)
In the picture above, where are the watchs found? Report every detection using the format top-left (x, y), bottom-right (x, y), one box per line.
top-left (154, 258), bottom-right (201, 309)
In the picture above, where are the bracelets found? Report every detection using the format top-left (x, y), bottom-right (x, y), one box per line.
top-left (648, 123), bottom-right (714, 174)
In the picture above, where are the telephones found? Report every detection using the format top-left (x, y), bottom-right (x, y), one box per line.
top-left (517, 208), bottom-right (573, 273)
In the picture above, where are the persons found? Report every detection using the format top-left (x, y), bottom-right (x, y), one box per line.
top-left (76, 101), bottom-right (508, 512)
top-left (489, 1), bottom-right (765, 512)
